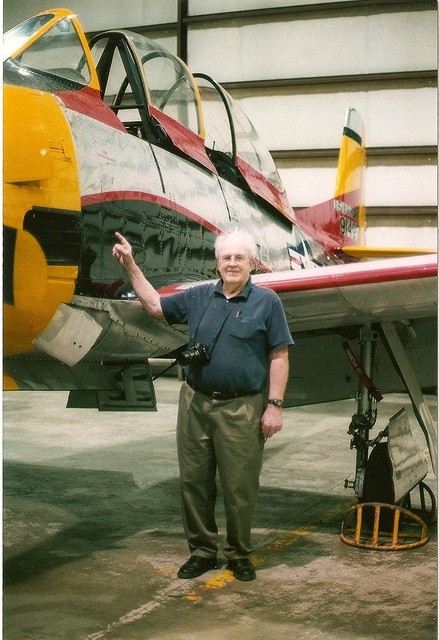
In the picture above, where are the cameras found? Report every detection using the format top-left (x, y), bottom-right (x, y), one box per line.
top-left (176, 343), bottom-right (211, 366)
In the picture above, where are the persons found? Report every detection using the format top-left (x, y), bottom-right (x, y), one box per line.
top-left (112, 229), bottom-right (294, 582)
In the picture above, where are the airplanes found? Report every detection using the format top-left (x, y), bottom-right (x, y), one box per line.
top-left (3, 6), bottom-right (438, 525)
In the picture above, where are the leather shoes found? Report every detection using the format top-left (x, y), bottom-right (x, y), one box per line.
top-left (227, 558), bottom-right (256, 581)
top-left (178, 555), bottom-right (219, 580)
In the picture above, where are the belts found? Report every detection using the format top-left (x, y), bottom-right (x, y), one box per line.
top-left (185, 379), bottom-right (259, 400)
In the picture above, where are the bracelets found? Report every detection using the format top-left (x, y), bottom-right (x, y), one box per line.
top-left (268, 399), bottom-right (283, 407)
